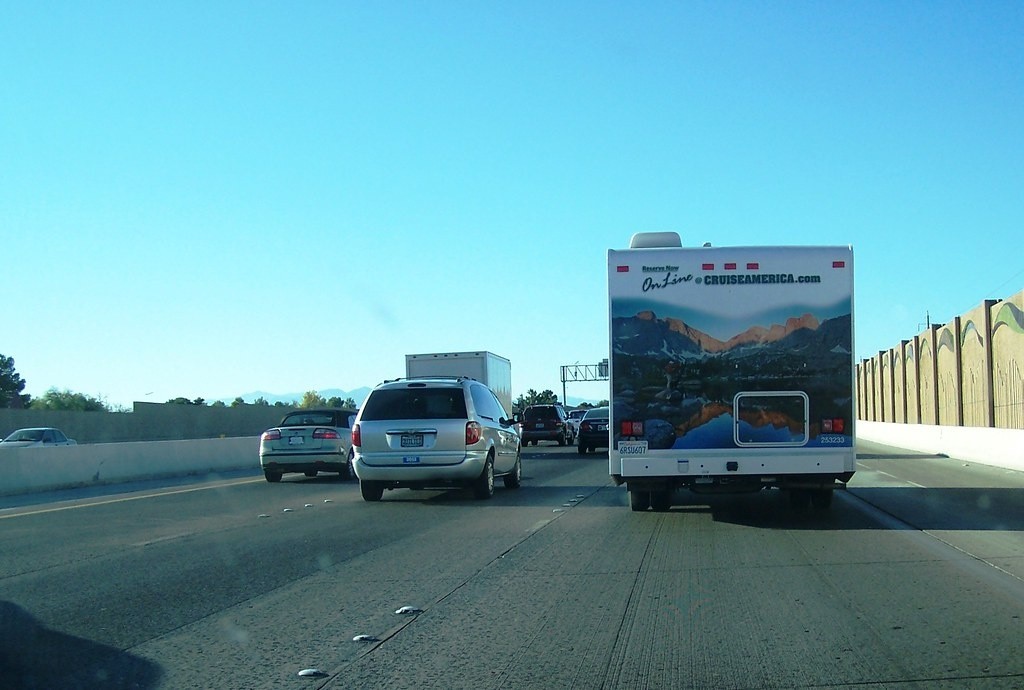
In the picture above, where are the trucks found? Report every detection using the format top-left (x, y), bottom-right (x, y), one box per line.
top-left (404, 350), bottom-right (520, 436)
top-left (606, 231), bottom-right (856, 513)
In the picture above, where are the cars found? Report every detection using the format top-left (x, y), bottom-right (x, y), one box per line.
top-left (260, 408), bottom-right (358, 483)
top-left (568, 409), bottom-right (588, 429)
top-left (574, 406), bottom-right (611, 455)
top-left (0, 429), bottom-right (78, 448)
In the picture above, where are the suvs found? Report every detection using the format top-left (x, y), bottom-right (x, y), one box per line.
top-left (352, 376), bottom-right (523, 502)
top-left (520, 402), bottom-right (576, 447)
